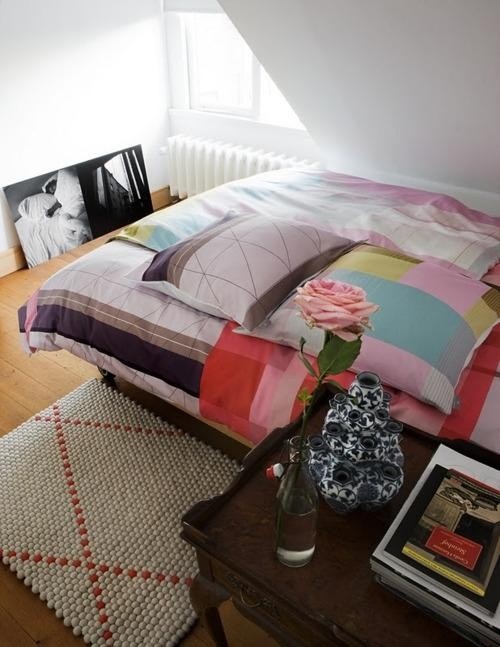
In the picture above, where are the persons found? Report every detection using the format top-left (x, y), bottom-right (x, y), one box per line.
top-left (43, 179), bottom-right (62, 218)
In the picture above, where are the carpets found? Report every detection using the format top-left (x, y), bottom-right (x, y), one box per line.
top-left (0, 374), bottom-right (245, 646)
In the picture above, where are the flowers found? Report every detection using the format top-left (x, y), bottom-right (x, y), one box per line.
top-left (275, 268), bottom-right (379, 530)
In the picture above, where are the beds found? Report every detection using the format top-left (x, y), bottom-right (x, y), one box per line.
top-left (26, 167), bottom-right (500, 468)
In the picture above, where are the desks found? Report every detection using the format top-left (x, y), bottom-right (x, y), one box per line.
top-left (164, 385), bottom-right (500, 647)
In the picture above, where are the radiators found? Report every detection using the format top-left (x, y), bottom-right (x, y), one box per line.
top-left (164, 134), bottom-right (290, 203)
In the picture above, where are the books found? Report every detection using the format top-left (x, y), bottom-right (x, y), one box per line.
top-left (370, 443), bottom-right (500, 647)
